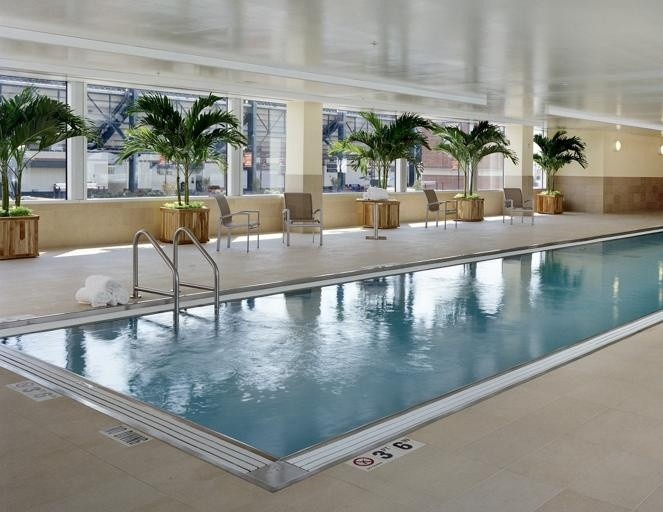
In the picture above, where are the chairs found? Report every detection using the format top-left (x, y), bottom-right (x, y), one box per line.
top-left (208, 188), bottom-right (260, 254)
top-left (499, 186), bottom-right (535, 226)
top-left (422, 190), bottom-right (459, 231)
top-left (280, 192), bottom-right (324, 246)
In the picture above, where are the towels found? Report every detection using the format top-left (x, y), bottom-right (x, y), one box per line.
top-left (73, 273), bottom-right (129, 308)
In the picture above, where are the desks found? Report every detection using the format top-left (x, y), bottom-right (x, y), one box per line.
top-left (355, 199), bottom-right (400, 241)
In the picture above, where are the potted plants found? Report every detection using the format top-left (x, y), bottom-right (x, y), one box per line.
top-left (0, 87), bottom-right (104, 259)
top-left (328, 111), bottom-right (516, 228)
top-left (532, 127), bottom-right (588, 216)
top-left (112, 91), bottom-right (247, 245)
